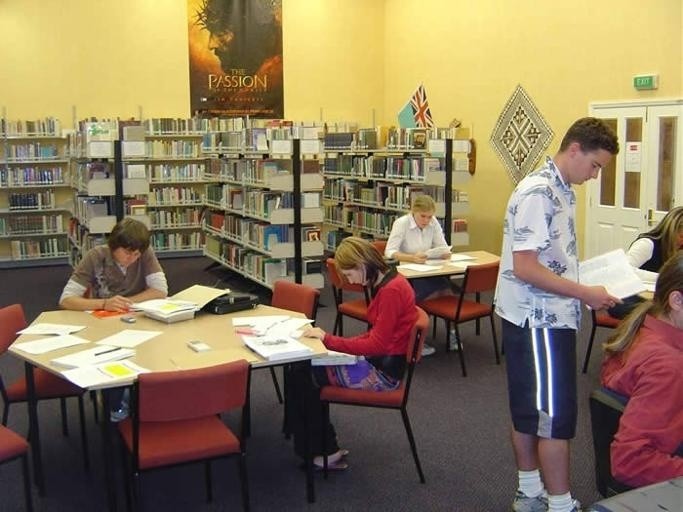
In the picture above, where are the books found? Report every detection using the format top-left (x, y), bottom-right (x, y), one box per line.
top-left (243, 333), bottom-right (313, 363)
top-left (323, 126), bottom-right (469, 250)
top-left (0, 113), bottom-right (476, 325)
top-left (70, 115), bottom-right (144, 269)
top-left (2, 116), bottom-right (71, 260)
top-left (310, 348), bottom-right (357, 366)
top-left (143, 117), bottom-right (322, 284)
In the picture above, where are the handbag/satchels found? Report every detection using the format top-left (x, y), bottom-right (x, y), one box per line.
top-left (199, 287), bottom-right (260, 314)
top-left (381, 253), bottom-right (400, 267)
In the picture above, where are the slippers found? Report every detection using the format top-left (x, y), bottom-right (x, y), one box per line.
top-left (296, 448), bottom-right (350, 473)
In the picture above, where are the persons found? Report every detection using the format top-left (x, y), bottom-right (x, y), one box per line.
top-left (383, 194), bottom-right (464, 359)
top-left (55, 214), bottom-right (168, 422)
top-left (205, 0), bottom-right (282, 109)
top-left (598, 250), bottom-right (682, 489)
top-left (605, 205), bottom-right (683, 320)
top-left (279, 235), bottom-right (419, 472)
top-left (489, 117), bottom-right (625, 512)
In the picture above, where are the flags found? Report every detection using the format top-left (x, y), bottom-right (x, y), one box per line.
top-left (396, 83), bottom-right (432, 129)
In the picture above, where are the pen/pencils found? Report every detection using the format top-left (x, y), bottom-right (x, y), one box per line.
top-left (94, 347), bottom-right (122, 356)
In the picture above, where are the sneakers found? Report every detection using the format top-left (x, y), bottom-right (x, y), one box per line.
top-left (110, 401), bottom-right (129, 422)
top-left (420, 343), bottom-right (438, 357)
top-left (448, 330), bottom-right (464, 352)
top-left (571, 499), bottom-right (583, 512)
top-left (507, 490), bottom-right (548, 512)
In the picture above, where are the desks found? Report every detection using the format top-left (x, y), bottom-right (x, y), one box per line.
top-left (396, 247), bottom-right (502, 336)
top-left (6, 303), bottom-right (326, 512)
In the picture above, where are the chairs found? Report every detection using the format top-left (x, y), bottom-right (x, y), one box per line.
top-left (265, 280), bottom-right (321, 404)
top-left (0, 304), bottom-right (97, 512)
top-left (583, 307), bottom-right (623, 373)
top-left (588, 384), bottom-right (683, 496)
top-left (105, 359), bottom-right (252, 512)
top-left (325, 258), bottom-right (374, 338)
top-left (316, 306), bottom-right (430, 485)
top-left (371, 239), bottom-right (437, 339)
top-left (416, 259), bottom-right (500, 377)
